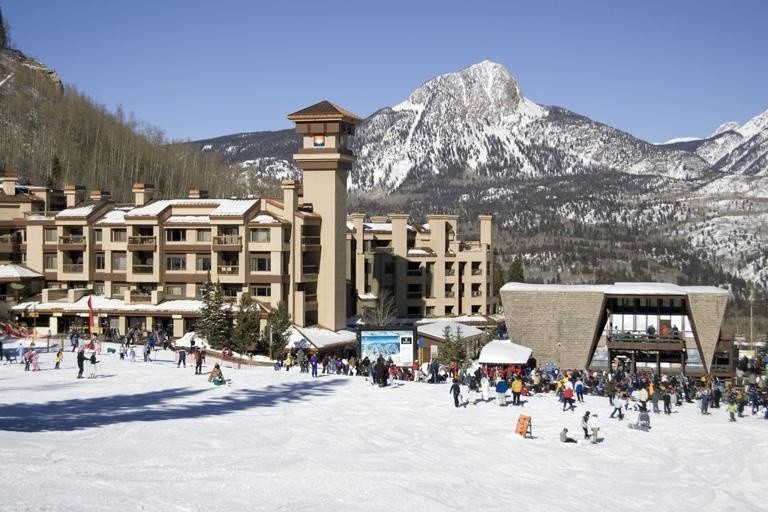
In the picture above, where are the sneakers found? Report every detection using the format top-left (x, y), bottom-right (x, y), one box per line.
top-left (24, 367), bottom-right (41, 371)
top-left (195, 371), bottom-right (203, 375)
top-left (76, 373), bottom-right (96, 379)
top-left (602, 402), bottom-right (768, 437)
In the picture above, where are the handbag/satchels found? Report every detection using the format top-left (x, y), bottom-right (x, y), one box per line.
top-left (213, 378), bottom-right (224, 386)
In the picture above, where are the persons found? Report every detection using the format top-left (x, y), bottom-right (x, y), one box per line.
top-left (581, 411), bottom-right (591, 438)
top-left (0, 324), bottom-right (101, 379)
top-left (118, 326), bottom-right (232, 374)
top-left (612, 325), bottom-right (679, 342)
top-left (208, 363), bottom-right (226, 385)
top-left (589, 414), bottom-right (600, 443)
top-left (560, 429), bottom-right (577, 443)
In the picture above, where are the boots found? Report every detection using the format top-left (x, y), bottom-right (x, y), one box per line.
top-left (563, 406), bottom-right (575, 411)
top-left (499, 399), bottom-right (521, 406)
top-left (176, 364), bottom-right (186, 368)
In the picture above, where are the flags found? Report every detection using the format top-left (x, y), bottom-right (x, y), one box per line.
top-left (88, 297), bottom-right (94, 327)
